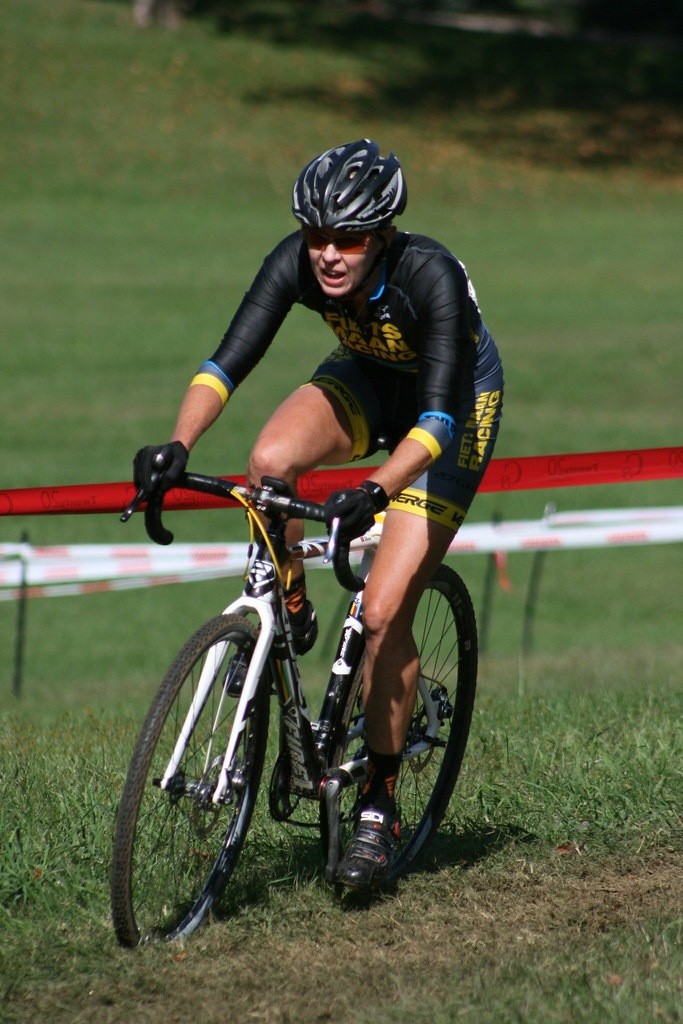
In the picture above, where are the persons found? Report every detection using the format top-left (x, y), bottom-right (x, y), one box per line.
top-left (133, 137), bottom-right (504, 890)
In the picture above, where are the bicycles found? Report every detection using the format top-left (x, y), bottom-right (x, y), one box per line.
top-left (111, 446), bottom-right (482, 950)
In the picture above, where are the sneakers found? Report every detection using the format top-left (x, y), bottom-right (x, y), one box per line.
top-left (220, 598), bottom-right (318, 696)
top-left (335, 803), bottom-right (402, 891)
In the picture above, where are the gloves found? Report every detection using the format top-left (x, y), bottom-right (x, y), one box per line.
top-left (324, 489), bottom-right (377, 539)
top-left (133, 442), bottom-right (186, 494)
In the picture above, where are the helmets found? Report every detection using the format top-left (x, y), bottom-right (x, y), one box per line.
top-left (291, 137), bottom-right (408, 231)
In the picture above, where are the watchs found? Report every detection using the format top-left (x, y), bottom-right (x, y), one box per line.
top-left (358, 480), bottom-right (390, 515)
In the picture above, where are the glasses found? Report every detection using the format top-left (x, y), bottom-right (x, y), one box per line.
top-left (305, 233), bottom-right (366, 254)
